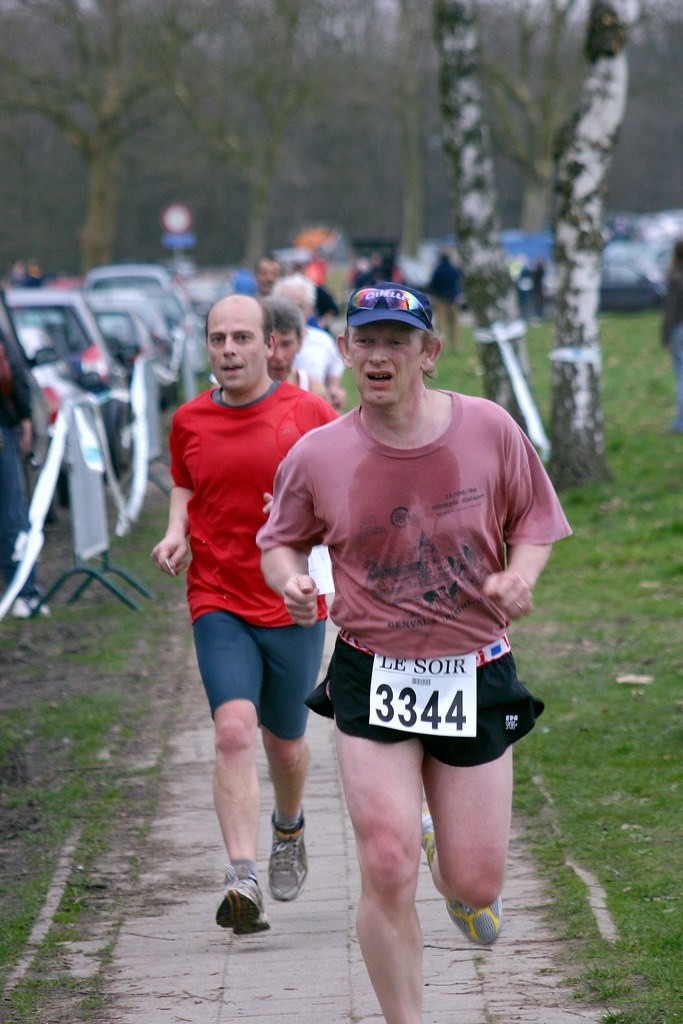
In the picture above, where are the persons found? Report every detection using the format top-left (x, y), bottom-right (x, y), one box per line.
top-left (9, 259), bottom-right (55, 288)
top-left (151, 294), bottom-right (341, 935)
top-left (659, 240), bottom-right (683, 433)
top-left (253, 250), bottom-right (393, 414)
top-left (429, 253), bottom-right (462, 302)
top-left (255, 282), bottom-right (572, 1024)
top-left (0, 329), bottom-right (50, 618)
top-left (509, 254), bottom-right (544, 321)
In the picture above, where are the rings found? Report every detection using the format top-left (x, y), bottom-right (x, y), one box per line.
top-left (516, 602), bottom-right (523, 610)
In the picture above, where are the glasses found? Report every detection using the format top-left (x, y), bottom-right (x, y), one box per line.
top-left (348, 287), bottom-right (431, 328)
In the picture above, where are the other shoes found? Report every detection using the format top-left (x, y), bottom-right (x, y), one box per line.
top-left (10, 595), bottom-right (50, 618)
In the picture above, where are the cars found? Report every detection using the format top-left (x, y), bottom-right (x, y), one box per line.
top-left (6, 210), bottom-right (683, 524)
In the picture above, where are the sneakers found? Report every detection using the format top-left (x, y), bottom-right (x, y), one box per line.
top-left (421, 800), bottom-right (503, 946)
top-left (215, 864), bottom-right (271, 935)
top-left (266, 809), bottom-right (309, 902)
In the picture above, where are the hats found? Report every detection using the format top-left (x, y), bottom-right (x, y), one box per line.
top-left (347, 282), bottom-right (432, 331)
